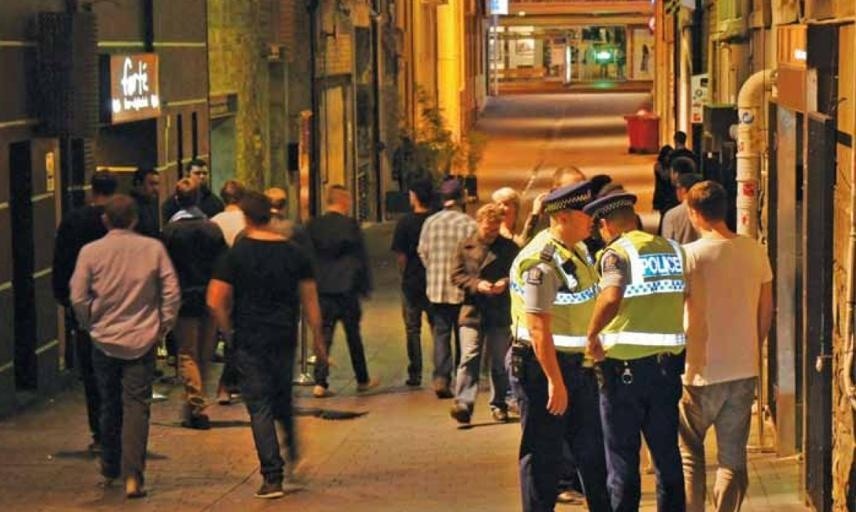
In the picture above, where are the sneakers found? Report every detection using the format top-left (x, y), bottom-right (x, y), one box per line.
top-left (180, 414), bottom-right (211, 429)
top-left (313, 384), bottom-right (325, 397)
top-left (556, 489), bottom-right (584, 505)
top-left (356, 377), bottom-right (380, 392)
top-left (124, 475), bottom-right (146, 498)
top-left (405, 377), bottom-right (521, 423)
top-left (253, 482), bottom-right (284, 499)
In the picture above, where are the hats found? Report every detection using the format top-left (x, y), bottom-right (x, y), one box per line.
top-left (583, 193), bottom-right (637, 224)
top-left (440, 180), bottom-right (461, 200)
top-left (542, 180), bottom-right (596, 214)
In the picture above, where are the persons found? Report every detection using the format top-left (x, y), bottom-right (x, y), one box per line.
top-left (304, 186), bottom-right (382, 396)
top-left (584, 193), bottom-right (689, 512)
top-left (67, 194), bottom-right (182, 497)
top-left (502, 178), bottom-right (614, 512)
top-left (204, 189), bottom-right (342, 501)
top-left (665, 173), bottom-right (703, 245)
top-left (391, 164), bottom-right (645, 423)
top-left (675, 178), bottom-right (776, 512)
top-left (651, 144), bottom-right (675, 211)
top-left (669, 128), bottom-right (700, 207)
top-left (51, 159), bottom-right (320, 430)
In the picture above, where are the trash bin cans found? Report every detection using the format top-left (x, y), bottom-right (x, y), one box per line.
top-left (623, 112), bottom-right (660, 154)
